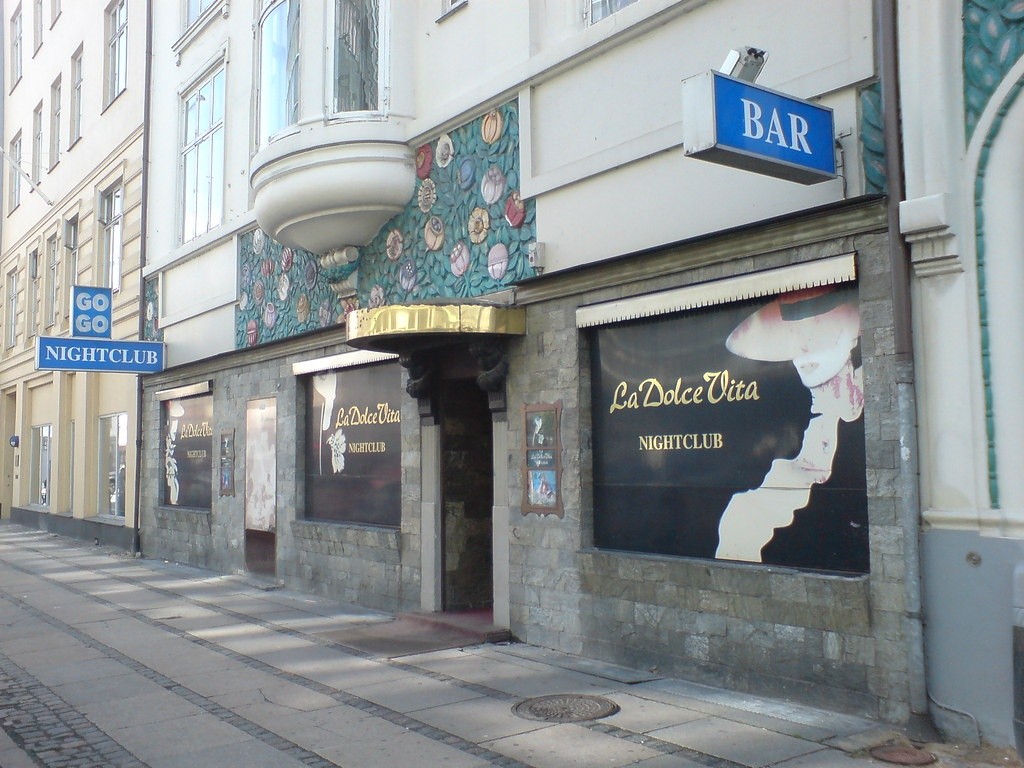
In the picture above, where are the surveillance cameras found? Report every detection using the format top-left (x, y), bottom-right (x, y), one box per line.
top-left (719, 46), bottom-right (765, 84)
